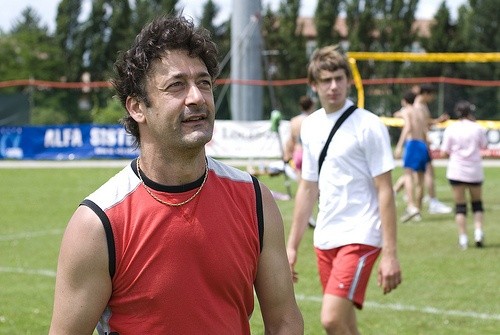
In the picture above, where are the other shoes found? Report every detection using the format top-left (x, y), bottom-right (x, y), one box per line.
top-left (475, 230), bottom-right (484, 247)
top-left (308, 218), bottom-right (316, 228)
top-left (428, 202), bottom-right (452, 214)
top-left (403, 207), bottom-right (420, 222)
top-left (460, 239), bottom-right (468, 251)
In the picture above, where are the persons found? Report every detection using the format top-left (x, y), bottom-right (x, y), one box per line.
top-left (392, 84), bottom-right (452, 223)
top-left (49, 15), bottom-right (305, 334)
top-left (287, 45), bottom-right (401, 334)
top-left (283, 96), bottom-right (316, 228)
top-left (440, 102), bottom-right (487, 249)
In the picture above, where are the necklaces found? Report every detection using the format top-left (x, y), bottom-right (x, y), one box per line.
top-left (137, 156), bottom-right (208, 206)
